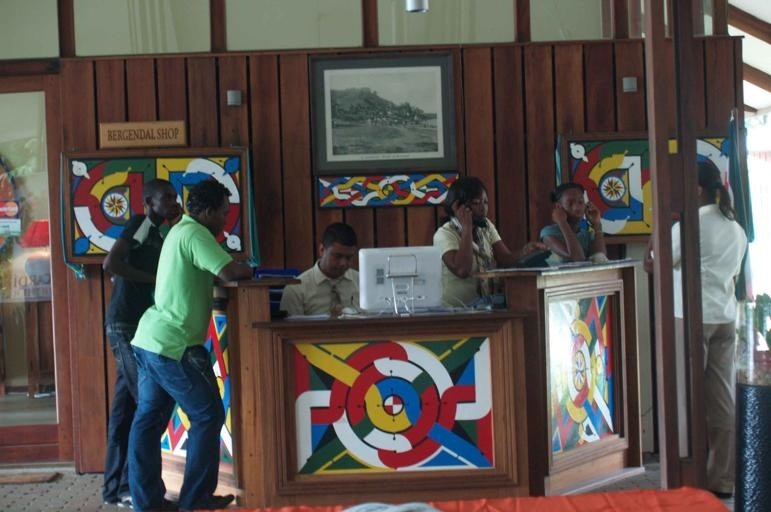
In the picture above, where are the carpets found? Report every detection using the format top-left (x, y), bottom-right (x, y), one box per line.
top-left (0, 470), bottom-right (62, 485)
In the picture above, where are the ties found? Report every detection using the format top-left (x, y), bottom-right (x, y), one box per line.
top-left (328, 281), bottom-right (343, 317)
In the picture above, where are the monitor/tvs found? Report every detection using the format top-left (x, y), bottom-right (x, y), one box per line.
top-left (358, 246), bottom-right (443, 316)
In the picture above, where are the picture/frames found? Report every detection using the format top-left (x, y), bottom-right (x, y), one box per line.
top-left (668, 129), bottom-right (742, 224)
top-left (59, 149), bottom-right (252, 264)
top-left (560, 131), bottom-right (653, 245)
top-left (308, 51), bottom-right (458, 176)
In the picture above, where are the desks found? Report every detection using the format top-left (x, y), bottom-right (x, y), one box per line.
top-left (24, 253), bottom-right (56, 398)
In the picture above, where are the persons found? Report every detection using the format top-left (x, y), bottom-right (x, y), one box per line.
top-left (127, 178), bottom-right (254, 512)
top-left (278, 222), bottom-right (359, 317)
top-left (432, 177), bottom-right (514, 307)
top-left (540, 182), bottom-right (608, 261)
top-left (102, 178), bottom-right (186, 507)
top-left (644, 153), bottom-right (749, 499)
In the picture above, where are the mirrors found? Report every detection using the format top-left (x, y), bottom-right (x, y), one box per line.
top-left (0, 88), bottom-right (60, 428)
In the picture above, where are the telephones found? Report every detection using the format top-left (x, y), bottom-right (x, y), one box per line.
top-left (448, 199), bottom-right (488, 227)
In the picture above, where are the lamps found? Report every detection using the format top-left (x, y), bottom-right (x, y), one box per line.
top-left (21, 218), bottom-right (50, 256)
top-left (405, 0), bottom-right (429, 14)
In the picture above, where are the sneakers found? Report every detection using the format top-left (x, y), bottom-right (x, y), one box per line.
top-left (104, 491), bottom-right (234, 512)
